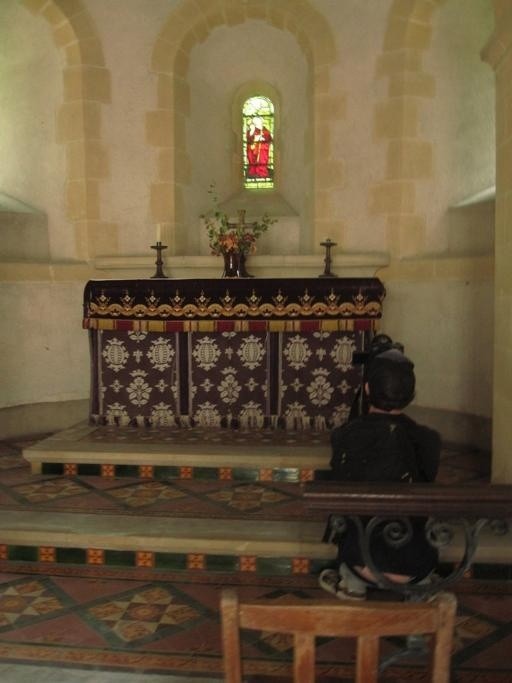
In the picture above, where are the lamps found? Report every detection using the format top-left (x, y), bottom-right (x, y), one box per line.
top-left (215, 586), bottom-right (461, 681)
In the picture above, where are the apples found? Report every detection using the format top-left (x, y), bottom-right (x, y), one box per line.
top-left (156, 221), bottom-right (162, 243)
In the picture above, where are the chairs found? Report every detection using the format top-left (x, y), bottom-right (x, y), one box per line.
top-left (319, 567), bottom-right (368, 602)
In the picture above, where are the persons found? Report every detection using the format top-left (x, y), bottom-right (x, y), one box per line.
top-left (316, 342), bottom-right (445, 605)
top-left (245, 116), bottom-right (276, 179)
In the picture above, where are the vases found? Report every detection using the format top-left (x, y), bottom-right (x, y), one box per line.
top-left (198, 178), bottom-right (281, 256)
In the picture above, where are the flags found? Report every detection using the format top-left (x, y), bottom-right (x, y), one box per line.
top-left (222, 251), bottom-right (252, 278)
